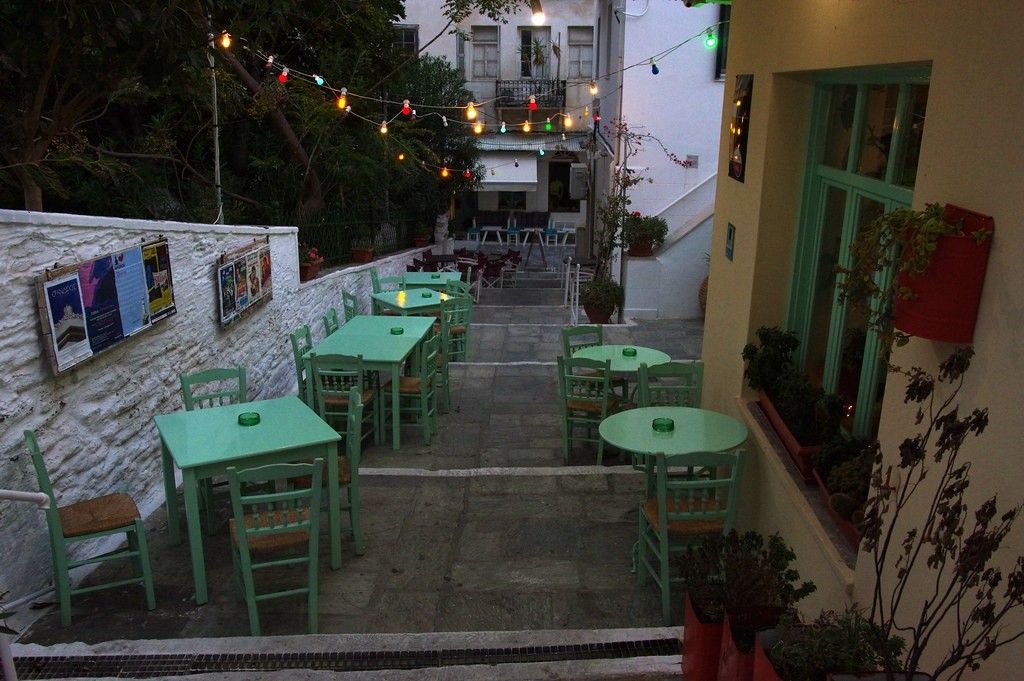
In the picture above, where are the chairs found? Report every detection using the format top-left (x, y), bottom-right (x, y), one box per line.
top-left (20, 209), bottom-right (749, 635)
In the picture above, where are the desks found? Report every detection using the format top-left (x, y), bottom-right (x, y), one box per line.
top-left (488, 255), bottom-right (501, 261)
top-left (572, 344), bottom-right (672, 400)
top-left (370, 288), bottom-right (457, 317)
top-left (429, 254), bottom-right (458, 267)
top-left (336, 314), bottom-right (437, 420)
top-left (482, 226), bottom-right (503, 247)
top-left (399, 272), bottom-right (462, 292)
top-left (598, 406), bottom-right (748, 573)
top-left (562, 257), bottom-right (595, 269)
top-left (523, 227), bottom-right (545, 246)
top-left (561, 228), bottom-right (575, 246)
top-left (303, 331), bottom-right (421, 451)
top-left (154, 394), bottom-right (342, 608)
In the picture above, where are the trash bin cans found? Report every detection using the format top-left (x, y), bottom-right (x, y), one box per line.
top-left (515, 211), bottom-right (549, 242)
top-left (483, 210), bottom-right (511, 240)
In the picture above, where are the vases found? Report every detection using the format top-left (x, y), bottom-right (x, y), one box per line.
top-left (629, 240), bottom-right (653, 256)
top-left (299, 259), bottom-right (323, 284)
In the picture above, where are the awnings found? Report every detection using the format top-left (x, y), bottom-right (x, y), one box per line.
top-left (473, 151), bottom-right (538, 192)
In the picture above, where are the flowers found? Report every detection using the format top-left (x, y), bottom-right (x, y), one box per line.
top-left (618, 210), bottom-right (669, 247)
top-left (298, 243), bottom-right (324, 264)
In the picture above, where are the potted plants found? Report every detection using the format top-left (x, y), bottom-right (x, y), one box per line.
top-left (579, 276), bottom-right (625, 324)
top-left (830, 204), bottom-right (994, 356)
top-left (413, 222), bottom-right (434, 248)
top-left (679, 325), bottom-right (902, 680)
top-left (351, 244), bottom-right (374, 264)
